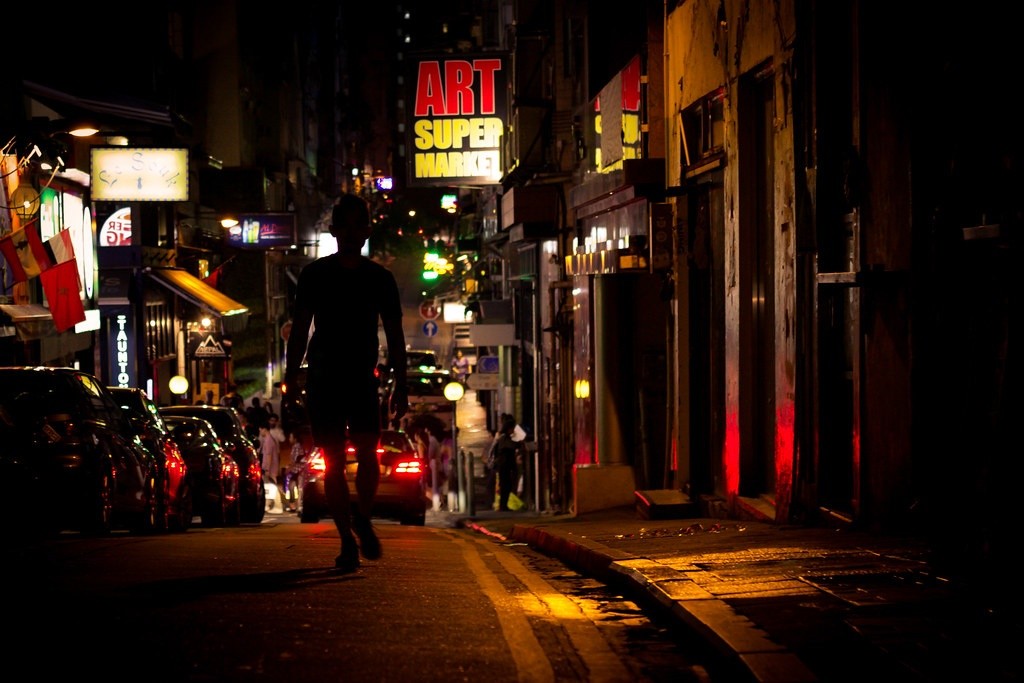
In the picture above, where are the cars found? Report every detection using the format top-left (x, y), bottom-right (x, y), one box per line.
top-left (300, 430), bottom-right (428, 527)
top-left (0, 367), bottom-right (161, 531)
top-left (106, 388), bottom-right (191, 530)
top-left (278, 365), bottom-right (309, 423)
top-left (158, 406), bottom-right (266, 525)
top-left (162, 413), bottom-right (240, 523)
top-left (389, 370), bottom-right (457, 433)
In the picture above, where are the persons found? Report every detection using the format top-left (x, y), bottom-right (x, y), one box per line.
top-left (196, 390), bottom-right (310, 516)
top-left (282, 191), bottom-right (407, 570)
top-left (381, 347), bottom-right (530, 515)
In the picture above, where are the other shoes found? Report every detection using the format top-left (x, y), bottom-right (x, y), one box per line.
top-left (336, 537), bottom-right (360, 568)
top-left (351, 512), bottom-right (381, 560)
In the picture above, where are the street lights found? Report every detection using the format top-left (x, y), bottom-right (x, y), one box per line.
top-left (168, 376), bottom-right (188, 409)
top-left (442, 383), bottom-right (465, 512)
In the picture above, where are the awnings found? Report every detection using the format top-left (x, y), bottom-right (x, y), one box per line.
top-left (420, 248), bottom-right (501, 307)
top-left (151, 265), bottom-right (248, 316)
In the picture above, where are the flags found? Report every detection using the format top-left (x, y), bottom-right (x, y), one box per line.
top-left (1, 221), bottom-right (52, 289)
top-left (38, 258), bottom-right (85, 334)
top-left (42, 228), bottom-right (82, 292)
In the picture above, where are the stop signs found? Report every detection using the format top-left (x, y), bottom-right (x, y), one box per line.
top-left (281, 323), bottom-right (293, 339)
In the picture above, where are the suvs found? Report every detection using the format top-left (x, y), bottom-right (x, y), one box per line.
top-left (403, 350), bottom-right (444, 372)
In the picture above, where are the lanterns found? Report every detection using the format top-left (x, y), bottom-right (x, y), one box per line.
top-left (11, 180), bottom-right (40, 222)
